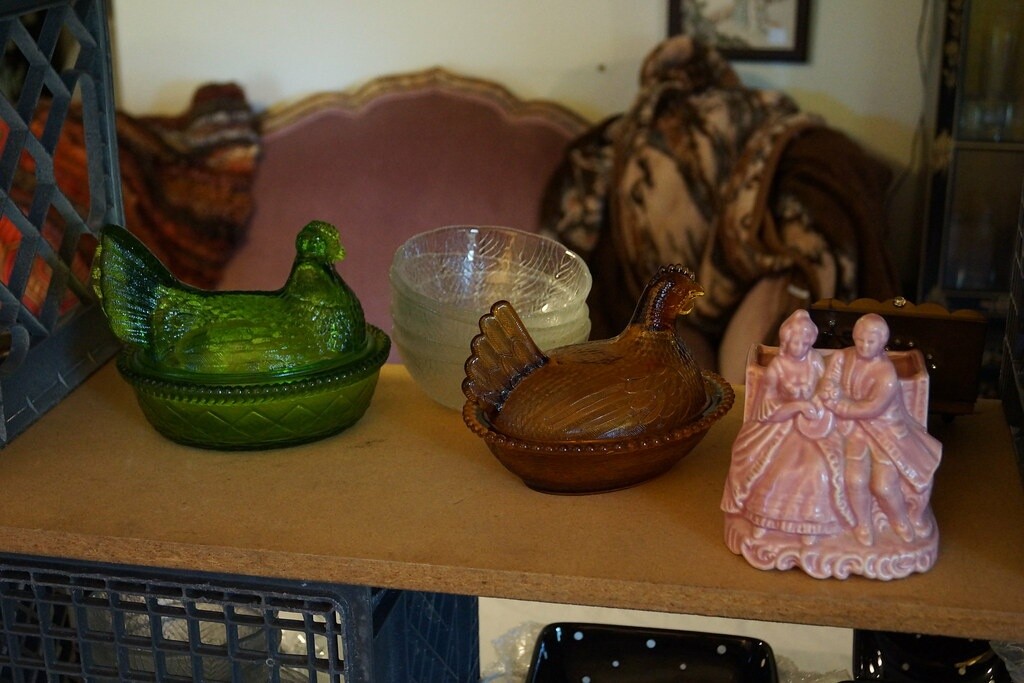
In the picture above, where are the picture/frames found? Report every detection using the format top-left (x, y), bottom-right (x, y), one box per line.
top-left (668, 0), bottom-right (812, 65)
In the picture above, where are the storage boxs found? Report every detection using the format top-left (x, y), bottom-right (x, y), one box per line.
top-left (0, 552), bottom-right (482, 683)
top-left (0, 0), bottom-right (128, 452)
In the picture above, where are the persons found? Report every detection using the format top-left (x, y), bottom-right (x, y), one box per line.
top-left (722, 312), bottom-right (943, 546)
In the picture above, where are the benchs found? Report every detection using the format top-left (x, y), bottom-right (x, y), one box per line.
top-left (224, 69), bottom-right (594, 359)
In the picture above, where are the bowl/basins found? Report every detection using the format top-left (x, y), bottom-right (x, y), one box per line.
top-left (386, 225), bottom-right (592, 409)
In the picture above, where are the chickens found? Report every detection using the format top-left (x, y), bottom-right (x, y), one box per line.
top-left (90, 224), bottom-right (367, 372)
top-left (460, 263), bottom-right (706, 443)
